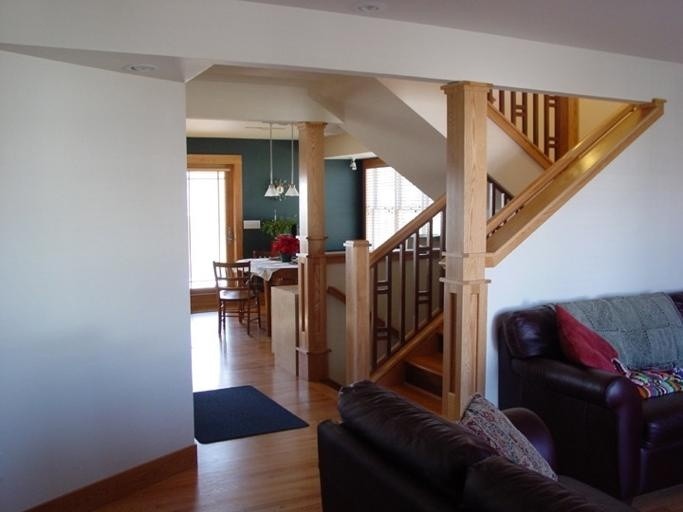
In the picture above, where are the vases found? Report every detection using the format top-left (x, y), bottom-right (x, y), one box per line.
top-left (280, 252), bottom-right (291, 263)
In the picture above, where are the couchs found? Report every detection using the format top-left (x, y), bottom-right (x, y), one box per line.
top-left (316, 378), bottom-right (637, 512)
top-left (498, 291), bottom-right (683, 505)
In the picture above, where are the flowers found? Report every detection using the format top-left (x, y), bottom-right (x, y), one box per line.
top-left (273, 234), bottom-right (301, 255)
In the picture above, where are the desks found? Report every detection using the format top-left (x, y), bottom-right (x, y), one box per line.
top-left (236, 257), bottom-right (299, 337)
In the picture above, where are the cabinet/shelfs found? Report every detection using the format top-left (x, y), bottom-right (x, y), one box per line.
top-left (271, 285), bottom-right (300, 376)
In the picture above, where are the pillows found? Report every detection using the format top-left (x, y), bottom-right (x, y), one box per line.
top-left (458, 393), bottom-right (560, 482)
top-left (555, 305), bottom-right (622, 377)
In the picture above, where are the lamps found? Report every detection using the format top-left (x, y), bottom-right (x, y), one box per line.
top-left (350, 158), bottom-right (358, 170)
top-left (285, 123), bottom-right (299, 196)
top-left (264, 121), bottom-right (280, 197)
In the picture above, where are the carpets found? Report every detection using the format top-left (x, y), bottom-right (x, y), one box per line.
top-left (192, 385), bottom-right (309, 444)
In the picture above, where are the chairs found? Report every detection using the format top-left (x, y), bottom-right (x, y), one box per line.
top-left (213, 260), bottom-right (261, 335)
top-left (251, 250), bottom-right (278, 306)
